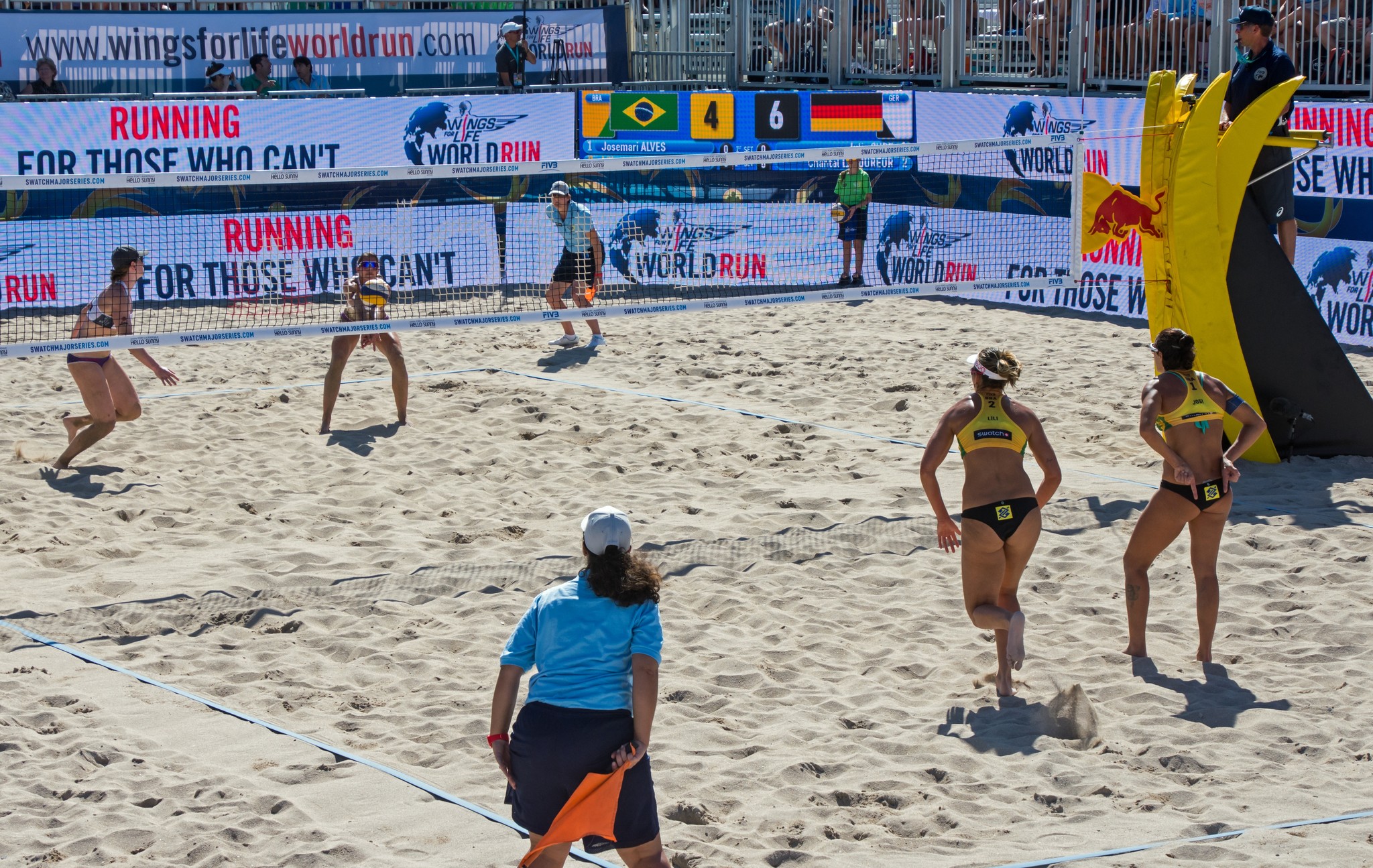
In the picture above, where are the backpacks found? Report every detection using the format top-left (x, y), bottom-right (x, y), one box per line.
top-left (747, 45), bottom-right (772, 82)
top-left (1320, 47), bottom-right (1353, 98)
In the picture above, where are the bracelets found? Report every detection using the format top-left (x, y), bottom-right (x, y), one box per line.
top-left (525, 48), bottom-right (530, 54)
top-left (233, 80), bottom-right (239, 87)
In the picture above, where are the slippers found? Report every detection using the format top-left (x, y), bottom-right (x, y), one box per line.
top-left (905, 68), bottom-right (924, 74)
top-left (891, 68), bottom-right (907, 74)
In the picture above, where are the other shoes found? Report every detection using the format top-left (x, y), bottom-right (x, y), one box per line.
top-left (854, 59), bottom-right (861, 64)
top-left (863, 60), bottom-right (872, 69)
top-left (837, 273), bottom-right (851, 285)
top-left (850, 272), bottom-right (865, 286)
top-left (1041, 68), bottom-right (1061, 78)
top-left (1027, 66), bottom-right (1047, 77)
top-left (774, 61), bottom-right (788, 68)
top-left (1094, 69), bottom-right (1148, 79)
top-left (928, 65), bottom-right (941, 73)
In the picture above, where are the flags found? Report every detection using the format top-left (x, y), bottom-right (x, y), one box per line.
top-left (516, 760), bottom-right (631, 868)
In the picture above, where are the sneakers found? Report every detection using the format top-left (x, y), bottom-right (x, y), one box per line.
top-left (548, 335), bottom-right (579, 345)
top-left (585, 336), bottom-right (606, 348)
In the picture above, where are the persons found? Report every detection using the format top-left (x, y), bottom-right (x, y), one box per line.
top-left (19, 57), bottom-right (69, 102)
top-left (197, 61), bottom-right (247, 100)
top-left (289, 56), bottom-right (333, 98)
top-left (921, 347), bottom-right (1062, 696)
top-left (53, 246), bottom-right (179, 471)
top-left (239, 53), bottom-right (284, 99)
top-left (546, 181), bottom-right (606, 348)
top-left (833, 158), bottom-right (872, 288)
top-left (1121, 327), bottom-right (1267, 662)
top-left (1219, 5), bottom-right (1299, 271)
top-left (319, 253), bottom-right (408, 434)
top-left (759, 0), bottom-right (1373, 104)
top-left (490, 506), bottom-right (675, 868)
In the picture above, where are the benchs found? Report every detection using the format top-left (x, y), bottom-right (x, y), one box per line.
top-left (635, 4), bottom-right (1362, 98)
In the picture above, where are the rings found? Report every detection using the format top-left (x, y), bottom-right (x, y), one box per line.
top-left (524, 44), bottom-right (525, 46)
top-left (495, 22), bottom-right (536, 95)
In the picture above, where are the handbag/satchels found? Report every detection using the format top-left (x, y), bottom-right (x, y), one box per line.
top-left (790, 48), bottom-right (829, 84)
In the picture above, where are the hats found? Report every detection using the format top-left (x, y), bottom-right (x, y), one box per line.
top-left (1227, 5), bottom-right (1274, 26)
top-left (966, 351), bottom-right (1008, 380)
top-left (210, 63), bottom-right (233, 77)
top-left (548, 181), bottom-right (569, 195)
top-left (581, 505), bottom-right (631, 556)
top-left (502, 22), bottom-right (523, 35)
top-left (111, 245), bottom-right (149, 270)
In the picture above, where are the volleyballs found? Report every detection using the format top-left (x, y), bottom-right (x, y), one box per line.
top-left (359, 279), bottom-right (389, 308)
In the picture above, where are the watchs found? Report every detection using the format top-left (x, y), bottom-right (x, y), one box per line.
top-left (594, 272), bottom-right (603, 277)
top-left (487, 733), bottom-right (509, 747)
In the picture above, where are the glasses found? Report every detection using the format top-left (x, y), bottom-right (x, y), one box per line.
top-left (1236, 23), bottom-right (1251, 30)
top-left (1150, 343), bottom-right (1158, 352)
top-left (137, 257), bottom-right (143, 263)
top-left (509, 30), bottom-right (521, 34)
top-left (846, 158), bottom-right (856, 161)
top-left (357, 261), bottom-right (379, 268)
top-left (551, 193), bottom-right (564, 197)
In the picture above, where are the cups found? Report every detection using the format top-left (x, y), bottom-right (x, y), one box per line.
top-left (965, 54), bottom-right (970, 73)
top-left (0, 95), bottom-right (3, 103)
top-left (1018, 54), bottom-right (1028, 62)
top-left (992, 54), bottom-right (1000, 62)
top-left (764, 75), bottom-right (777, 83)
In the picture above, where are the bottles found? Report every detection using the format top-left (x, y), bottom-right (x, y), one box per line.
top-left (1141, 72), bottom-right (1148, 94)
top-left (900, 81), bottom-right (915, 86)
top-left (765, 61), bottom-right (772, 71)
